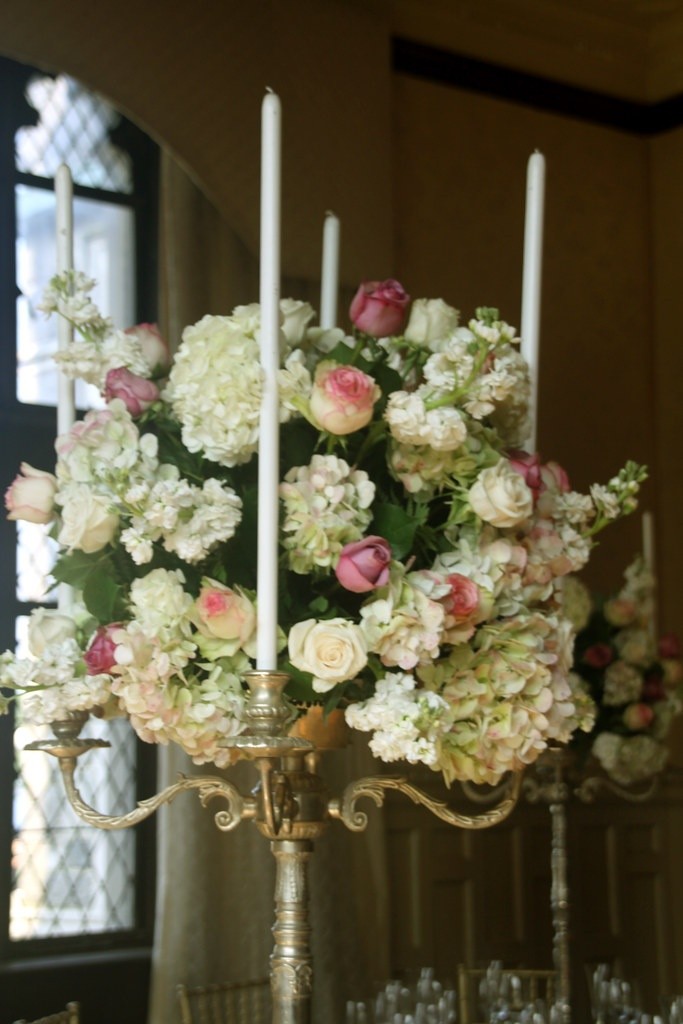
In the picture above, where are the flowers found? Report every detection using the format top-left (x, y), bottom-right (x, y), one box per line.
top-left (0, 265), bottom-right (649, 790)
top-left (569, 588), bottom-right (683, 784)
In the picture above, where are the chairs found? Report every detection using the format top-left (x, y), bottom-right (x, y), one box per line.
top-left (177, 976), bottom-right (274, 1024)
top-left (457, 962), bottom-right (571, 1024)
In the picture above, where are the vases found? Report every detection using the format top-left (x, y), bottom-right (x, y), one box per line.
top-left (288, 699), bottom-right (351, 752)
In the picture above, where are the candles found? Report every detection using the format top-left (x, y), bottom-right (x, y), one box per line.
top-left (641, 513), bottom-right (653, 572)
top-left (319, 213), bottom-right (342, 327)
top-left (55, 162), bottom-right (78, 436)
top-left (521, 149), bottom-right (547, 450)
top-left (256, 88), bottom-right (279, 671)
